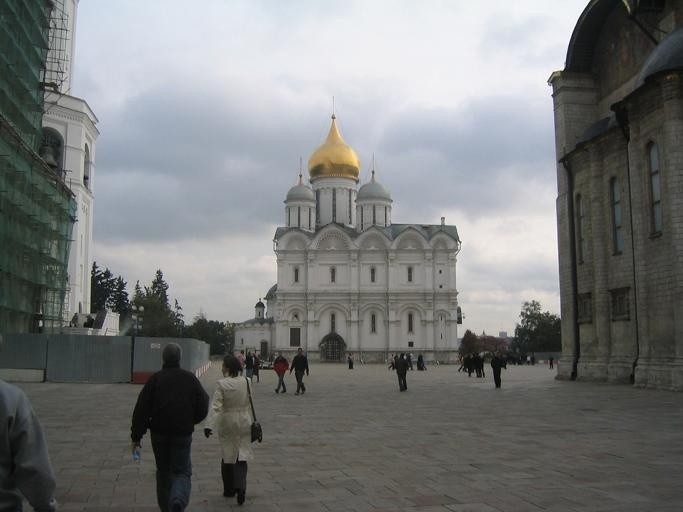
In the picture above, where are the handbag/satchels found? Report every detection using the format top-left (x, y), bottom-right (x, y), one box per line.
top-left (251, 421), bottom-right (262, 442)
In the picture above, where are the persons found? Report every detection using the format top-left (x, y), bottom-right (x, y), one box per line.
top-left (348, 352), bottom-right (353, 369)
top-left (234, 350), bottom-right (245, 376)
top-left (272, 350), bottom-right (288, 393)
top-left (0, 378), bottom-right (58, 511)
top-left (251, 353), bottom-right (260, 382)
top-left (129, 343), bottom-right (210, 511)
top-left (387, 349), bottom-right (557, 391)
top-left (203, 355), bottom-right (254, 504)
top-left (243, 351), bottom-right (253, 381)
top-left (288, 348), bottom-right (309, 395)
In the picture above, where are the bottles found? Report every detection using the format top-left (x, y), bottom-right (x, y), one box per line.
top-left (131, 446), bottom-right (141, 465)
